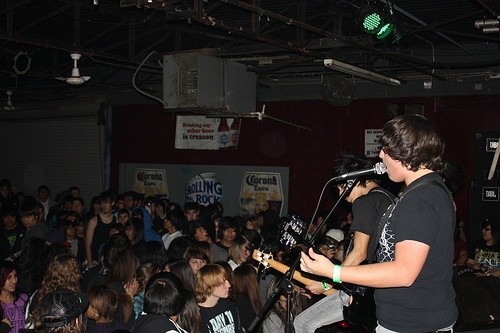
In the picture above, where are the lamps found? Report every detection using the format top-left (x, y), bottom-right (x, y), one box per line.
top-left (0, 48), bottom-right (31, 74)
top-left (350, 0), bottom-right (385, 34)
top-left (323, 40), bottom-right (401, 88)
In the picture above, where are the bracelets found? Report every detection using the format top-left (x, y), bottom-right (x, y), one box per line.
top-left (333, 265), bottom-right (342, 283)
top-left (321, 279), bottom-right (330, 291)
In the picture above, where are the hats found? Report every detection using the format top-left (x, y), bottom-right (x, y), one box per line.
top-left (242, 229), bottom-right (262, 248)
top-left (326, 229), bottom-right (344, 242)
top-left (40, 288), bottom-right (89, 327)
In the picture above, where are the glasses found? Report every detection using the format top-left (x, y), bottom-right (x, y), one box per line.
top-left (146, 202), bottom-right (155, 206)
top-left (324, 237), bottom-right (338, 246)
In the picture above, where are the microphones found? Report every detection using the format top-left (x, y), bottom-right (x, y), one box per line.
top-left (341, 162), bottom-right (387, 179)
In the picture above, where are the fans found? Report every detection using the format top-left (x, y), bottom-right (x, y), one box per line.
top-left (0, 52), bottom-right (158, 95)
top-left (0, 89), bottom-right (41, 113)
top-left (320, 76), bottom-right (354, 107)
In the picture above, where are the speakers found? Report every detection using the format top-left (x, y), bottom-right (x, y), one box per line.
top-left (468, 130), bottom-right (500, 252)
top-left (451, 275), bottom-right (500, 331)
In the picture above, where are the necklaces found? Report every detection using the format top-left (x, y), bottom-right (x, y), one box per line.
top-left (100, 212), bottom-right (113, 219)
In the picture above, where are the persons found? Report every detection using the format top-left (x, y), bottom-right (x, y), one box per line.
top-left (453, 220), bottom-right (500, 333)
top-left (293, 152), bottom-right (396, 333)
top-left (0, 179), bottom-right (355, 333)
top-left (300, 113), bottom-right (458, 333)
